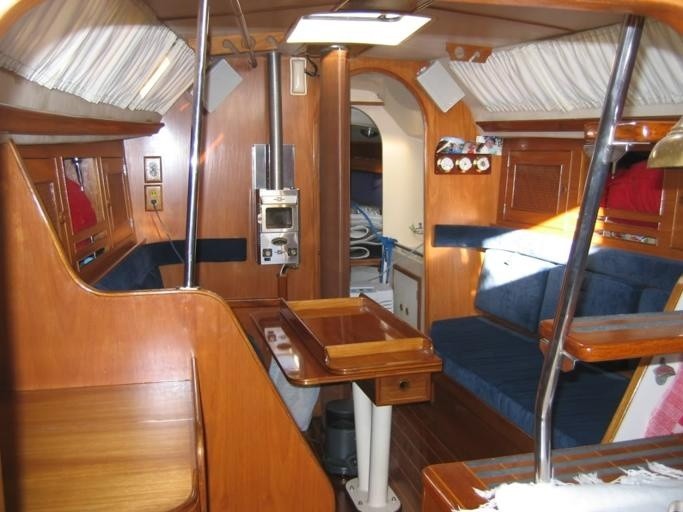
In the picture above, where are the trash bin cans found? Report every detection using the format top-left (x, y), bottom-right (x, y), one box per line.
top-left (323, 401), bottom-right (357, 476)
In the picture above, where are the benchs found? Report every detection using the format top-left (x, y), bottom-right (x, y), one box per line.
top-left (428, 224), bottom-right (683, 450)
top-left (89, 238), bottom-right (267, 371)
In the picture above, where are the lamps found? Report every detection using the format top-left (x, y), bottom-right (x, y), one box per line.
top-left (283, 10), bottom-right (436, 48)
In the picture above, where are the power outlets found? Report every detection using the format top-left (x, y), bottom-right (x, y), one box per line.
top-left (144, 185), bottom-right (163, 211)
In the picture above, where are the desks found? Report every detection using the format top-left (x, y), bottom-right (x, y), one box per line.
top-left (248, 292), bottom-right (442, 512)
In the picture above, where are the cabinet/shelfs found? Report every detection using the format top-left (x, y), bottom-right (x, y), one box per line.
top-left (416, 429), bottom-right (683, 512)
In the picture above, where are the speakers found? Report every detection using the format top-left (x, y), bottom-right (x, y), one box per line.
top-left (416, 60), bottom-right (466, 114)
top-left (187, 57), bottom-right (243, 113)
